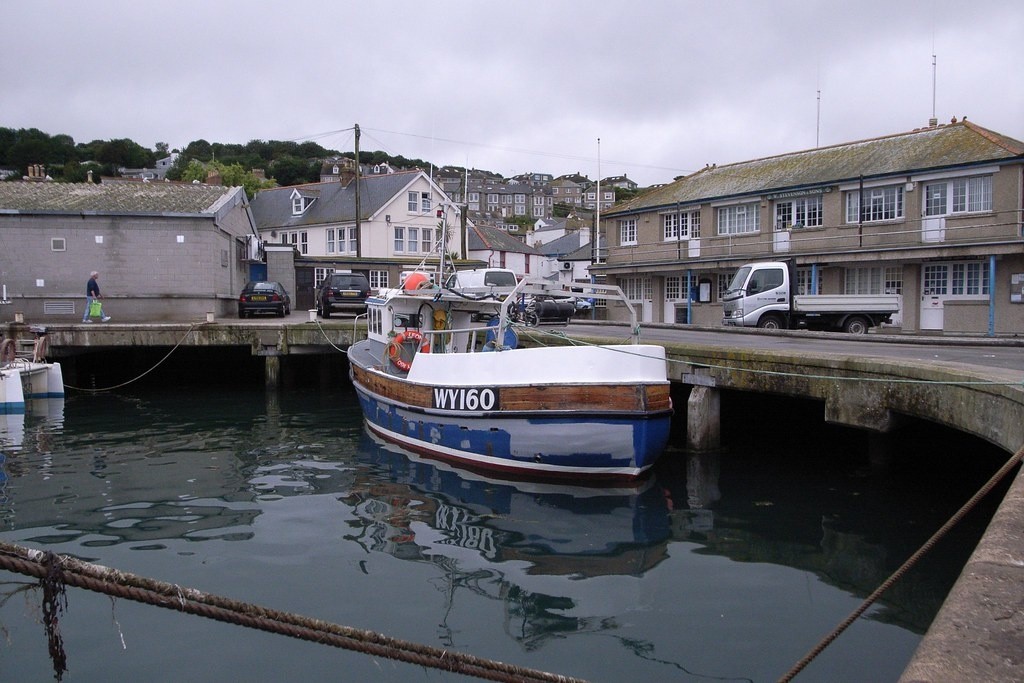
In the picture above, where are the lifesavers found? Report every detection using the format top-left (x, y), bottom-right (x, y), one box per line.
top-left (390, 331), bottom-right (430, 371)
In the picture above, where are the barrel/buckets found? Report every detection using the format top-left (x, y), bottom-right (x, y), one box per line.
top-left (535, 302), bottom-right (575, 317)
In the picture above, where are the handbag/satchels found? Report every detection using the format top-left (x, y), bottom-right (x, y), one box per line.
top-left (89, 299), bottom-right (101, 317)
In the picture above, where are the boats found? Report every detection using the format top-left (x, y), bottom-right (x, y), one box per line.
top-left (347, 202), bottom-right (678, 484)
top-left (0, 340), bottom-right (66, 406)
top-left (358, 417), bottom-right (678, 649)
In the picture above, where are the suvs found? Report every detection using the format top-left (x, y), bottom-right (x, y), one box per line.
top-left (315, 270), bottom-right (373, 319)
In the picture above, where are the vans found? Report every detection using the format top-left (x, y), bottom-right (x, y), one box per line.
top-left (442, 267), bottom-right (519, 323)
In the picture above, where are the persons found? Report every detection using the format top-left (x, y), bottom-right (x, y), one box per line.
top-left (82, 271), bottom-right (111, 323)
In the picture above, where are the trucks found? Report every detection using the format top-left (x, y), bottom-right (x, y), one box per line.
top-left (719, 254), bottom-right (902, 335)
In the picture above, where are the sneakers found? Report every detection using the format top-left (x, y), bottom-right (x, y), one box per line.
top-left (82, 319), bottom-right (93, 324)
top-left (101, 316), bottom-right (111, 323)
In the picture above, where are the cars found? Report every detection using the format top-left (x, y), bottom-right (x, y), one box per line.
top-left (519, 293), bottom-right (592, 310)
top-left (237, 280), bottom-right (292, 319)
